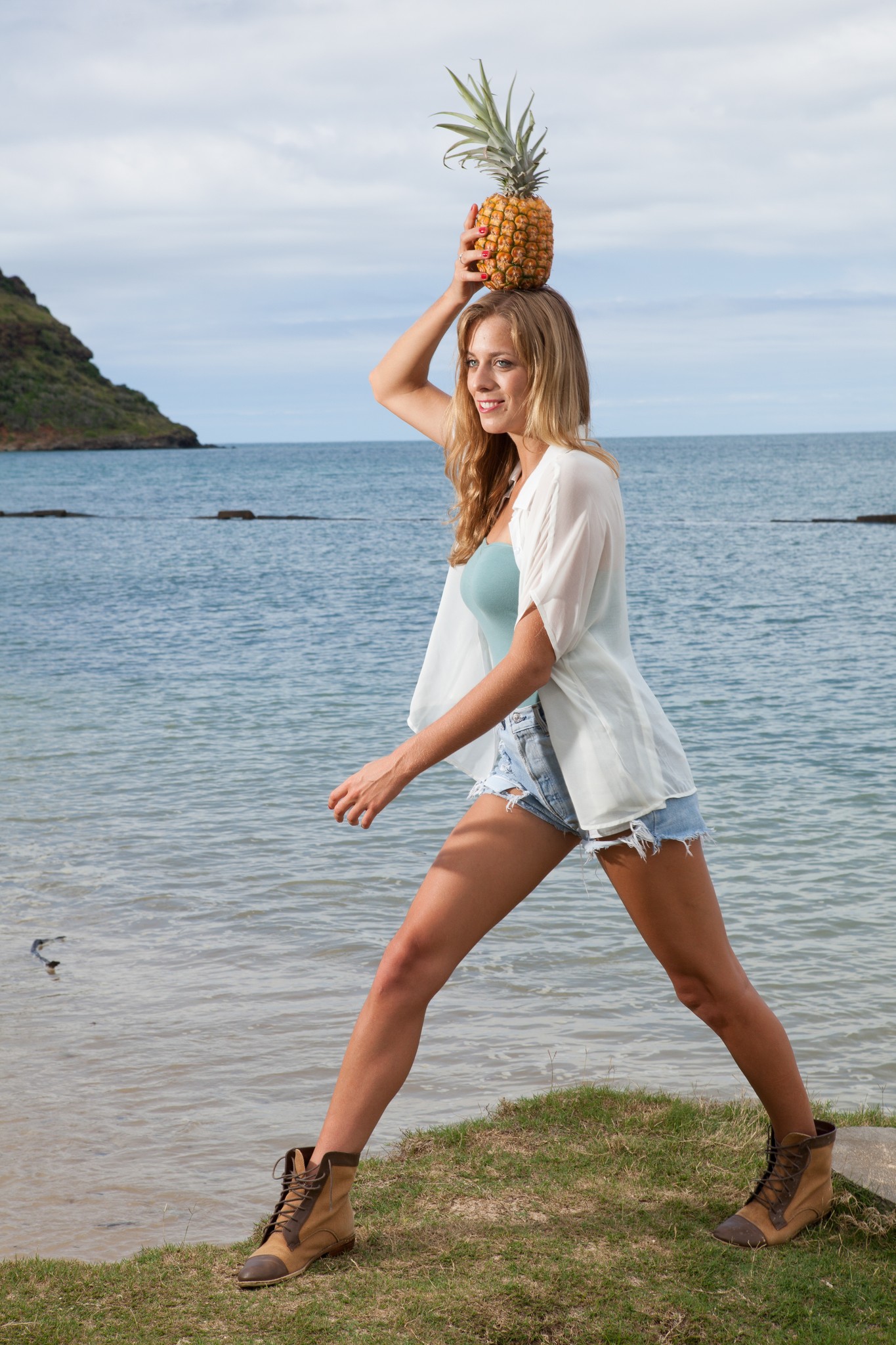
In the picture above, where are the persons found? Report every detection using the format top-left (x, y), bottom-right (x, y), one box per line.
top-left (235, 205), bottom-right (837, 1289)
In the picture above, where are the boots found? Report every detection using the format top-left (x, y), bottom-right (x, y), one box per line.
top-left (237, 1144), bottom-right (360, 1286)
top-left (712, 1120), bottom-right (837, 1251)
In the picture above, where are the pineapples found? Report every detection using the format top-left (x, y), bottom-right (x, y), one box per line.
top-left (433, 56), bottom-right (554, 291)
